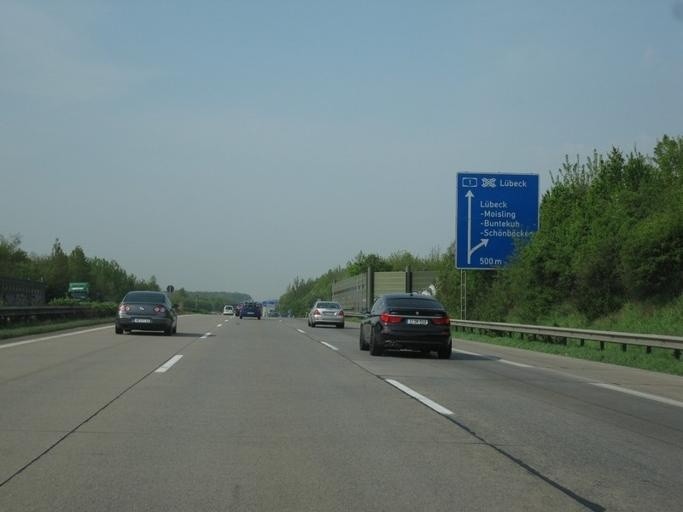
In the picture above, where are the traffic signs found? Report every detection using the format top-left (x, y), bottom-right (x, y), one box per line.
top-left (456, 171), bottom-right (540, 272)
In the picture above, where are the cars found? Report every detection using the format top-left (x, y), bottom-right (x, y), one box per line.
top-left (359, 294), bottom-right (453, 358)
top-left (308, 300), bottom-right (344, 329)
top-left (114, 290), bottom-right (180, 335)
top-left (222, 302), bottom-right (262, 319)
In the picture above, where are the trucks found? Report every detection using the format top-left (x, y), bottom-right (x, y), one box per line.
top-left (66, 281), bottom-right (102, 302)
